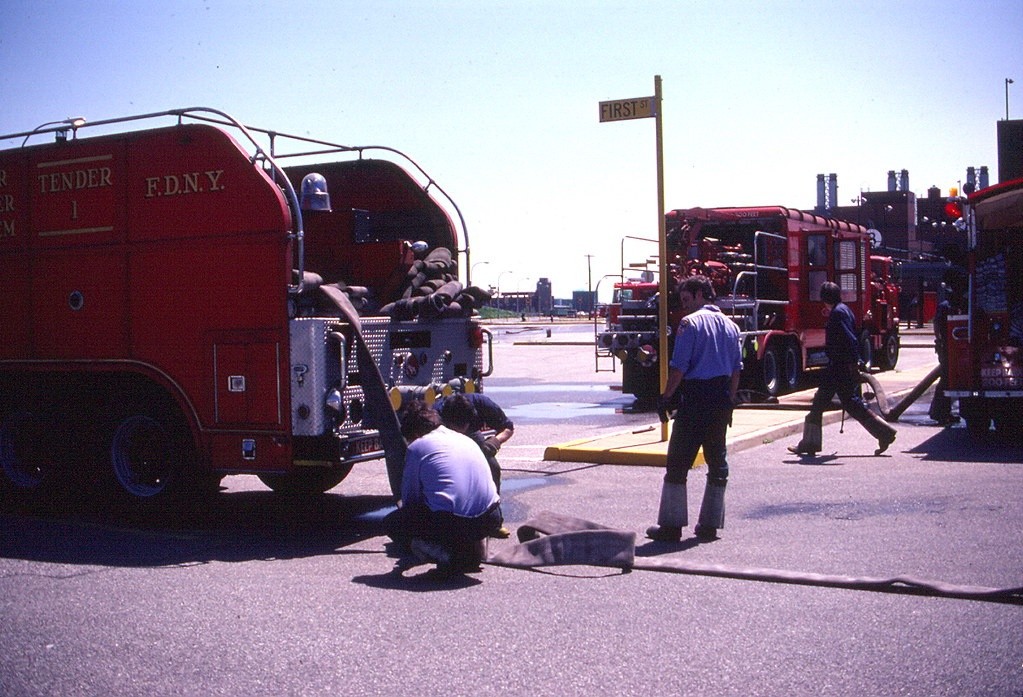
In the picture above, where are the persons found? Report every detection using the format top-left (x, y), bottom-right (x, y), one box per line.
top-left (927, 300), bottom-right (961, 423)
top-left (384, 399), bottom-right (503, 573)
top-left (647, 274), bottom-right (741, 543)
top-left (906, 291), bottom-right (919, 328)
top-left (787, 282), bottom-right (897, 456)
top-left (433, 394), bottom-right (514, 537)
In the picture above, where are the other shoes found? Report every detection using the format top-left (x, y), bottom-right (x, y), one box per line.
top-left (427, 556), bottom-right (452, 580)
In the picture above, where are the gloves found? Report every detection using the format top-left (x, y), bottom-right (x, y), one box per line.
top-left (482, 437), bottom-right (502, 458)
top-left (657, 393), bottom-right (675, 423)
top-left (727, 402), bottom-right (736, 428)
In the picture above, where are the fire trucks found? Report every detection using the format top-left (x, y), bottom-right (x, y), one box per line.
top-left (939, 178), bottom-right (1022, 455)
top-left (0, 106), bottom-right (495, 529)
top-left (594, 205), bottom-right (903, 412)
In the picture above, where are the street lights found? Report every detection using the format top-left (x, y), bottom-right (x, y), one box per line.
top-left (470, 261), bottom-right (489, 284)
top-left (1004, 77), bottom-right (1014, 120)
top-left (915, 215), bottom-right (938, 327)
top-left (517, 277), bottom-right (530, 319)
top-left (497, 270), bottom-right (512, 318)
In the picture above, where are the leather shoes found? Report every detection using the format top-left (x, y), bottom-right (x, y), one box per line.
top-left (875, 434), bottom-right (896, 455)
top-left (647, 525), bottom-right (681, 541)
top-left (787, 444), bottom-right (816, 456)
top-left (489, 527), bottom-right (510, 538)
top-left (695, 523), bottom-right (716, 540)
